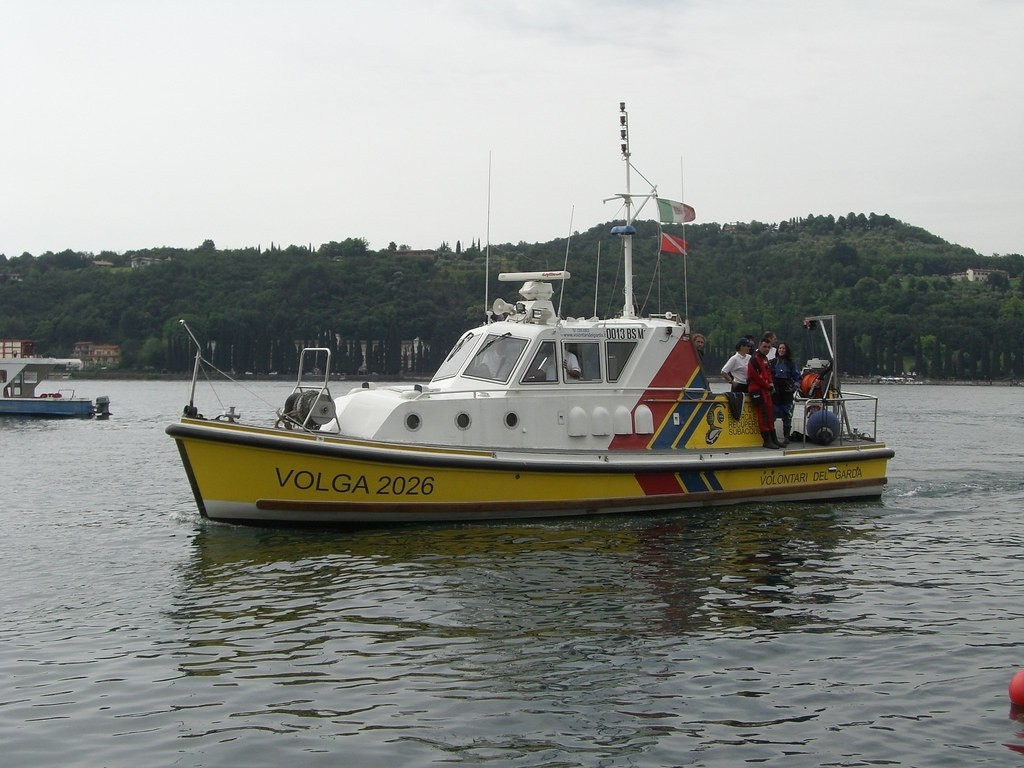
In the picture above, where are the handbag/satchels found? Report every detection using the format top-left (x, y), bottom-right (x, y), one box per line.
top-left (750, 390), bottom-right (763, 406)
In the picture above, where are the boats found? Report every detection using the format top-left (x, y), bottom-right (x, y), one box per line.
top-left (0, 356), bottom-right (113, 421)
top-left (164, 102), bottom-right (896, 534)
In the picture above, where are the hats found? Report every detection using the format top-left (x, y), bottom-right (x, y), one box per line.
top-left (736, 338), bottom-right (755, 346)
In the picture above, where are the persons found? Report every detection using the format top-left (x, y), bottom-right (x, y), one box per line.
top-left (720, 330), bottom-right (778, 392)
top-left (482, 341), bottom-right (511, 378)
top-left (693, 334), bottom-right (706, 361)
top-left (768, 342), bottom-right (802, 443)
top-left (533, 343), bottom-right (581, 380)
top-left (746, 337), bottom-right (787, 449)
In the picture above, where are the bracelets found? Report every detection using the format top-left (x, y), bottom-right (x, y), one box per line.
top-left (729, 380), bottom-right (733, 384)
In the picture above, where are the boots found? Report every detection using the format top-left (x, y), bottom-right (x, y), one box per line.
top-left (771, 430), bottom-right (787, 447)
top-left (783, 425), bottom-right (791, 443)
top-left (760, 430), bottom-right (779, 449)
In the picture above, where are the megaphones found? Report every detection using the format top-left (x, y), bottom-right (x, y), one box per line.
top-left (493, 298), bottom-right (514, 315)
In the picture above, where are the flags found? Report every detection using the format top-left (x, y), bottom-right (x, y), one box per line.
top-left (661, 233), bottom-right (688, 254)
top-left (656, 198), bottom-right (696, 223)
